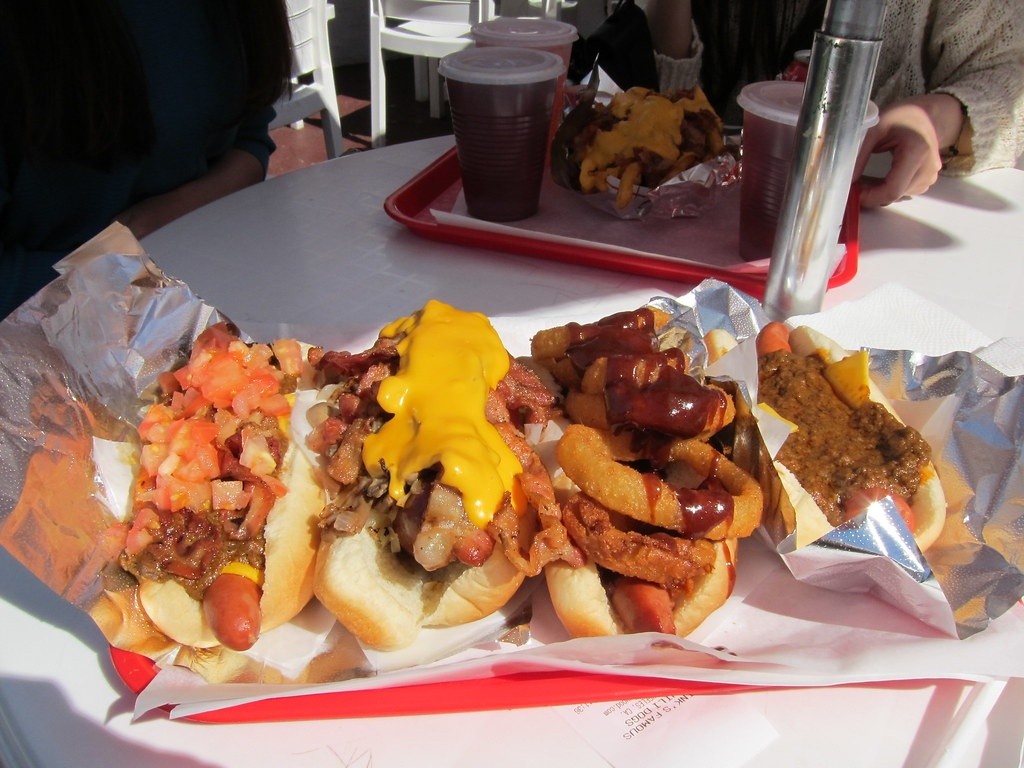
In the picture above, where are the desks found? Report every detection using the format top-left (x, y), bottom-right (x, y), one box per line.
top-left (0, 129), bottom-right (1024, 768)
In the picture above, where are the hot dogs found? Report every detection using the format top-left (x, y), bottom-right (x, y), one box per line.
top-left (700, 320), bottom-right (945, 559)
top-left (305, 299), bottom-right (541, 658)
top-left (514, 305), bottom-right (765, 640)
top-left (567, 83), bottom-right (723, 211)
top-left (117, 321), bottom-right (332, 652)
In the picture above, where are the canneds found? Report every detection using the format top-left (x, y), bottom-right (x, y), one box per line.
top-left (782, 48), bottom-right (811, 82)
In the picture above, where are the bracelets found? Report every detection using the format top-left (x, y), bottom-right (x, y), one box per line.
top-left (941, 105), bottom-right (968, 170)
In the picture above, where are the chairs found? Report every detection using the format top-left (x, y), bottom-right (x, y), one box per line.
top-left (368, 1), bottom-right (500, 149)
top-left (265, 0), bottom-right (344, 164)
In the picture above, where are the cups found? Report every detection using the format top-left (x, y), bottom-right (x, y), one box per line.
top-left (473, 18), bottom-right (580, 170)
top-left (438, 45), bottom-right (567, 222)
top-left (736, 79), bottom-right (880, 262)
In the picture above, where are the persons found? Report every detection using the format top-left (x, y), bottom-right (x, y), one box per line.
top-left (634, 0), bottom-right (1024, 209)
top-left (0, 0), bottom-right (293, 323)
